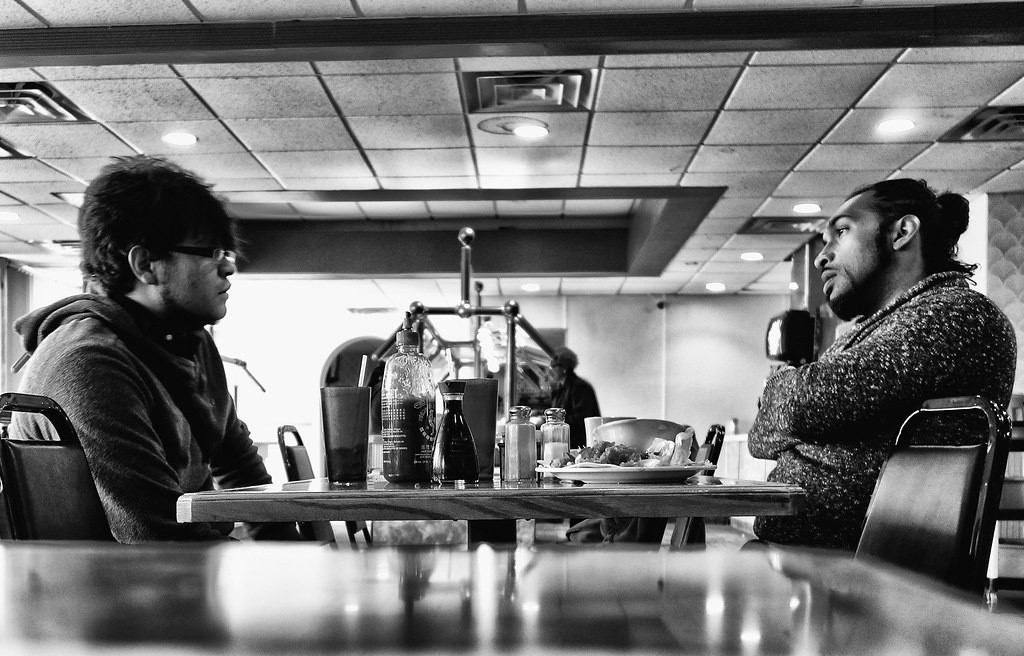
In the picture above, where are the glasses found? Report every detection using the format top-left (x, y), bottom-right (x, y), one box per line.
top-left (549, 363), bottom-right (568, 368)
top-left (168, 245), bottom-right (237, 265)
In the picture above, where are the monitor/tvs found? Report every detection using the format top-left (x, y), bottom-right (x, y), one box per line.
top-left (765, 310), bottom-right (815, 364)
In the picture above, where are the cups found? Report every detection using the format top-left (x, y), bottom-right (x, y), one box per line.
top-left (320, 386), bottom-right (372, 490)
top-left (583, 416), bottom-right (637, 447)
top-left (442, 377), bottom-right (498, 482)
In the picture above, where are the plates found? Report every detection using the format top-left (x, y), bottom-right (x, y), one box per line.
top-left (534, 458), bottom-right (717, 484)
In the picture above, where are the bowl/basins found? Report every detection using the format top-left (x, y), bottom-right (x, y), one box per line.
top-left (591, 418), bottom-right (685, 450)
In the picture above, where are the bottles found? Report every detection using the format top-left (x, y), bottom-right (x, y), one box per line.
top-left (542, 408), bottom-right (571, 478)
top-left (505, 405), bottom-right (536, 482)
top-left (438, 380), bottom-right (478, 482)
top-left (380, 312), bottom-right (436, 484)
top-left (497, 432), bottom-right (505, 480)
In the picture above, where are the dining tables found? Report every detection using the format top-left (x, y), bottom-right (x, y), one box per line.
top-left (0, 542), bottom-right (1024, 656)
top-left (175, 477), bottom-right (809, 553)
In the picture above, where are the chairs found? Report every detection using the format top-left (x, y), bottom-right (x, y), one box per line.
top-left (277, 425), bottom-right (338, 549)
top-left (0, 392), bottom-right (121, 543)
top-left (345, 521), bottom-right (372, 551)
top-left (668, 424), bottom-right (726, 548)
top-left (853, 393), bottom-right (1012, 607)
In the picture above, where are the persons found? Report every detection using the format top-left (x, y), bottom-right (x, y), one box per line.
top-left (741, 178), bottom-right (1018, 560)
top-left (4, 153), bottom-right (305, 545)
top-left (546, 347), bottom-right (602, 449)
